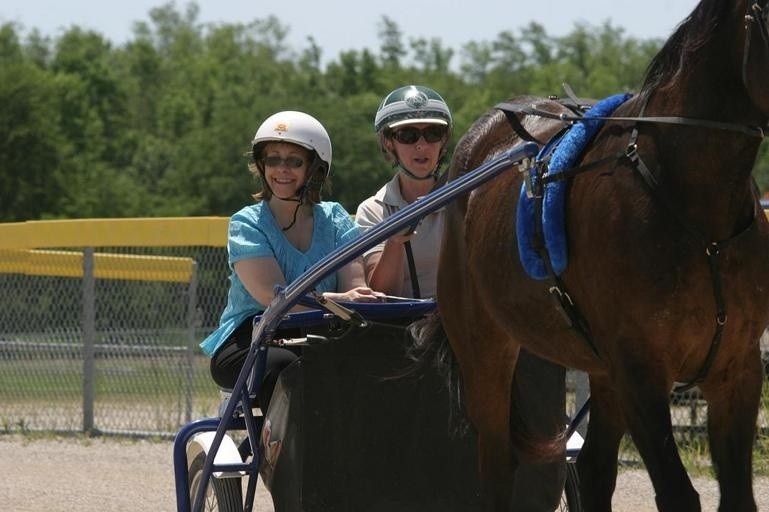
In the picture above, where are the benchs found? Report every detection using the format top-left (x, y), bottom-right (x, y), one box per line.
top-left (219, 388), bottom-right (257, 420)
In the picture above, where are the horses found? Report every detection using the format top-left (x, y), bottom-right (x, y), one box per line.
top-left (399, 0), bottom-right (769, 512)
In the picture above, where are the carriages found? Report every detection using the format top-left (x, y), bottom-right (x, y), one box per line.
top-left (172, 1), bottom-right (768, 512)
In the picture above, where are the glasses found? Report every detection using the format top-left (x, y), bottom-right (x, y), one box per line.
top-left (260, 155), bottom-right (308, 168)
top-left (387, 126), bottom-right (442, 145)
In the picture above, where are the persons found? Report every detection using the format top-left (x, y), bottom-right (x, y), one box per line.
top-left (351, 83), bottom-right (454, 303)
top-left (197, 109), bottom-right (387, 422)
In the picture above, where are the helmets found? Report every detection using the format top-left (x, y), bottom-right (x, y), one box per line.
top-left (376, 85), bottom-right (452, 136)
top-left (253, 109), bottom-right (332, 193)
